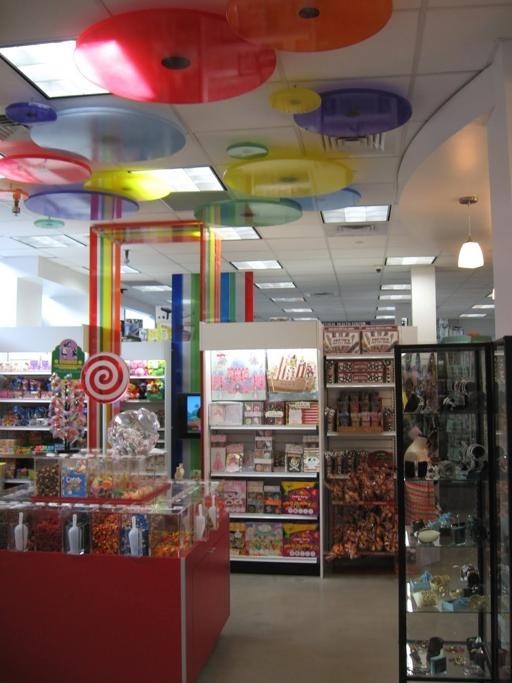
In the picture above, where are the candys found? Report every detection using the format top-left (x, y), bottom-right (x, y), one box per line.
top-left (0, 463), bottom-right (193, 556)
top-left (48, 372), bottom-right (84, 441)
top-left (81, 352), bottom-right (130, 403)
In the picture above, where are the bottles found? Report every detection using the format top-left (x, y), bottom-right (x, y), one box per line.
top-left (193, 502), bottom-right (205, 540)
top-left (207, 493), bottom-right (218, 529)
top-left (67, 514), bottom-right (84, 555)
top-left (128, 516), bottom-right (143, 556)
top-left (12, 512), bottom-right (29, 551)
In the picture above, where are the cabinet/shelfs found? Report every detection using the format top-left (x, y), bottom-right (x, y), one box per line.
top-left (196, 320), bottom-right (400, 579)
top-left (395, 335), bottom-right (510, 681)
top-left (0, 318), bottom-right (230, 681)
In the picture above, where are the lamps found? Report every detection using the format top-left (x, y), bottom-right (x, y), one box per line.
top-left (458, 196), bottom-right (486, 270)
